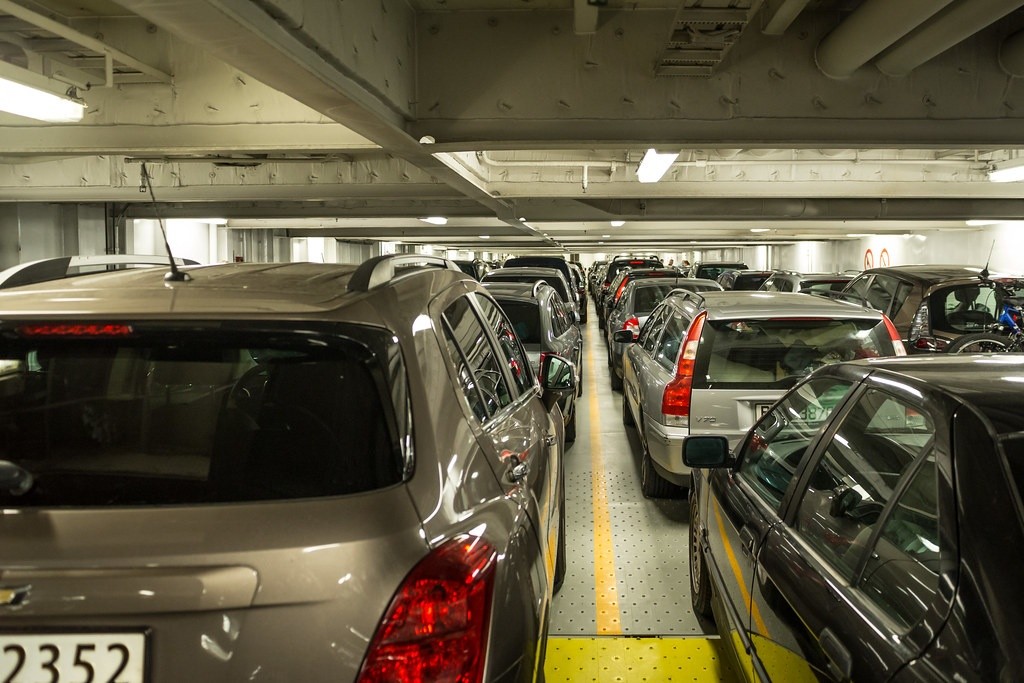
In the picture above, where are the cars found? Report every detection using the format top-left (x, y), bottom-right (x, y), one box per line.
top-left (683, 351), bottom-right (1024, 682)
top-left (606, 277), bottom-right (726, 390)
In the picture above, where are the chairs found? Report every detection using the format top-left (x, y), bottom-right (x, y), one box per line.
top-left (946, 286), bottom-right (994, 325)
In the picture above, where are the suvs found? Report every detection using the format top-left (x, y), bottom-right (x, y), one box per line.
top-left (472, 253), bottom-right (585, 440)
top-left (0, 252), bottom-right (576, 683)
top-left (713, 269), bottom-right (778, 292)
top-left (839, 263), bottom-right (1024, 354)
top-left (687, 261), bottom-right (749, 280)
top-left (613, 286), bottom-right (930, 503)
top-left (588, 255), bottom-right (686, 337)
top-left (755, 270), bottom-right (857, 300)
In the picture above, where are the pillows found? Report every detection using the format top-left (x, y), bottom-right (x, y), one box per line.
top-left (716, 337), bottom-right (787, 371)
top-left (706, 353), bottom-right (776, 382)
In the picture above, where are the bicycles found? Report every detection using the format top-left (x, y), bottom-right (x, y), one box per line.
top-left (945, 273), bottom-right (1024, 352)
